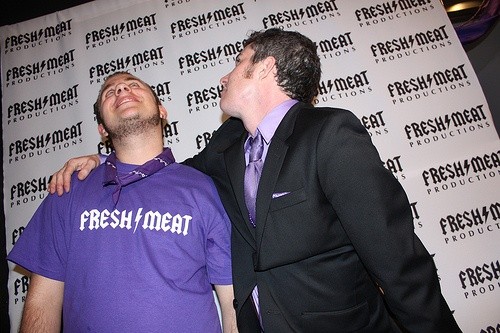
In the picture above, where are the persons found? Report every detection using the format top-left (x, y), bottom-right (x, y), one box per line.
top-left (5, 69), bottom-right (239, 333)
top-left (46, 27), bottom-right (462, 333)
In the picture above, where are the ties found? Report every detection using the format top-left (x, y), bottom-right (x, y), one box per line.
top-left (243, 130), bottom-right (264, 229)
